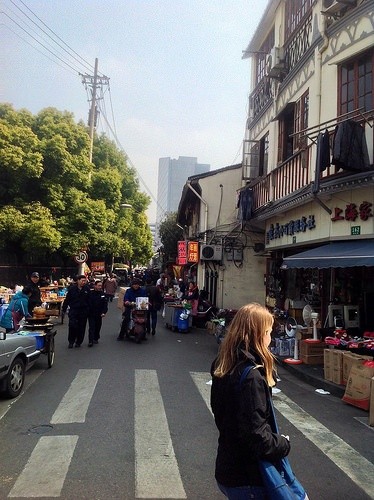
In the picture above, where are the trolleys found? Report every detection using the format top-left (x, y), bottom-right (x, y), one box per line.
top-left (165, 305), bottom-right (192, 332)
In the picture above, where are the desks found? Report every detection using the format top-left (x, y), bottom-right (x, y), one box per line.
top-left (287, 308), bottom-right (302, 319)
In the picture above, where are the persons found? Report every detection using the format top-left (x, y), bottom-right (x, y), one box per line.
top-left (85, 280), bottom-right (108, 346)
top-left (86, 265), bottom-right (199, 321)
top-left (61, 275), bottom-right (90, 348)
top-left (38, 275), bottom-right (75, 291)
top-left (25, 272), bottom-right (42, 317)
top-left (142, 278), bottom-right (163, 335)
top-left (116, 281), bottom-right (148, 341)
top-left (0, 285), bottom-right (34, 334)
top-left (209, 302), bottom-right (291, 500)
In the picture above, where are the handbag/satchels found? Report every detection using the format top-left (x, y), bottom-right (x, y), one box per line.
top-left (237, 365), bottom-right (311, 500)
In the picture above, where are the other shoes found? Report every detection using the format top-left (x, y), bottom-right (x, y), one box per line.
top-left (152, 330), bottom-right (155, 334)
top-left (75, 344), bottom-right (80, 347)
top-left (117, 333), bottom-right (123, 340)
top-left (89, 343), bottom-right (92, 346)
top-left (147, 328), bottom-right (150, 334)
top-left (93, 340), bottom-right (98, 344)
top-left (69, 344), bottom-right (73, 348)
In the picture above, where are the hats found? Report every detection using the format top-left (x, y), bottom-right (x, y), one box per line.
top-left (31, 272), bottom-right (39, 277)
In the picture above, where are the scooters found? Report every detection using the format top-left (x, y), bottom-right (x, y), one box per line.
top-left (123, 302), bottom-right (152, 343)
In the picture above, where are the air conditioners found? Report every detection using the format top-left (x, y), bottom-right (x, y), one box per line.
top-left (264, 46), bottom-right (285, 77)
top-left (200, 245), bottom-right (223, 261)
top-left (320, 0), bottom-right (347, 16)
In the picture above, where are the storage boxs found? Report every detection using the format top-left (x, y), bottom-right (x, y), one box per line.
top-left (117, 287), bottom-right (130, 310)
top-left (19, 332), bottom-right (44, 350)
top-left (324, 349), bottom-right (374, 410)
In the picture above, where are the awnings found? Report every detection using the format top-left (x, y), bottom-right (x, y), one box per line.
top-left (280, 242), bottom-right (374, 271)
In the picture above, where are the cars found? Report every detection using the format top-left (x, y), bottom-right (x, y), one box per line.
top-left (0, 332), bottom-right (42, 399)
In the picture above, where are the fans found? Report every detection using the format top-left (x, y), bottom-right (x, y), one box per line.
top-left (303, 304), bottom-right (320, 343)
top-left (283, 317), bottom-right (301, 364)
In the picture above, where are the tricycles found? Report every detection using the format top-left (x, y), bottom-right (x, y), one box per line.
top-left (45, 307), bottom-right (64, 324)
top-left (19, 329), bottom-right (57, 367)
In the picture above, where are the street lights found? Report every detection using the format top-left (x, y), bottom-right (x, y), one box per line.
top-left (118, 203), bottom-right (132, 209)
